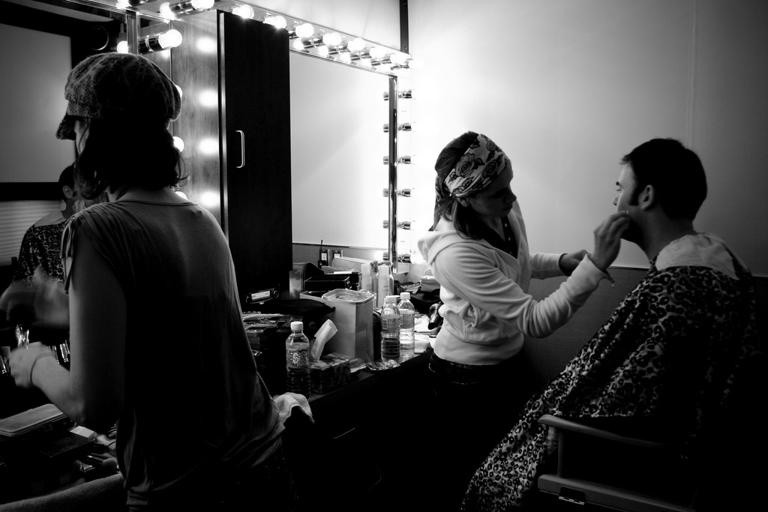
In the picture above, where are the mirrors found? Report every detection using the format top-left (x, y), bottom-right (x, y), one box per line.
top-left (0, 0), bottom-right (142, 418)
top-left (286, 45), bottom-right (399, 314)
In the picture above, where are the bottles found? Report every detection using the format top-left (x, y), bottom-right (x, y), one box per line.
top-left (396, 292), bottom-right (415, 350)
top-left (284, 321), bottom-right (312, 400)
top-left (380, 295), bottom-right (401, 362)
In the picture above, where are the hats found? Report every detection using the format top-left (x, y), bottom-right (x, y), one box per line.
top-left (53, 50), bottom-right (183, 141)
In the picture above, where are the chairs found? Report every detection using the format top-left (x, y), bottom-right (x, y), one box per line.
top-left (534, 271), bottom-right (767, 512)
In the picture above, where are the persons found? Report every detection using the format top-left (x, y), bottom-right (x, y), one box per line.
top-left (18, 161), bottom-right (109, 292)
top-left (1, 50), bottom-right (293, 510)
top-left (413, 129), bottom-right (630, 512)
top-left (593, 135), bottom-right (766, 431)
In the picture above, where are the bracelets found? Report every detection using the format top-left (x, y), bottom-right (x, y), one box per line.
top-left (29, 351), bottom-right (57, 385)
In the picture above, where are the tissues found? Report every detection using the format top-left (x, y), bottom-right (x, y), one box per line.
top-left (309, 318), bottom-right (352, 395)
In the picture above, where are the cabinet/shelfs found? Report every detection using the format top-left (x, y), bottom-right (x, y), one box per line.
top-left (168, 5), bottom-right (294, 306)
